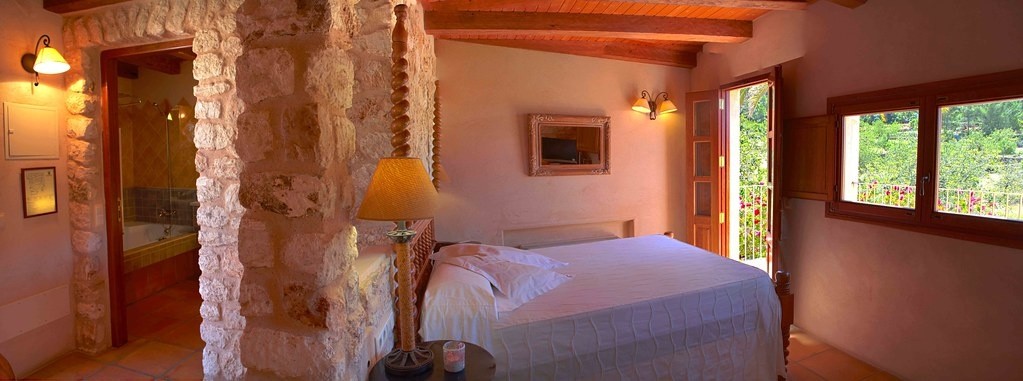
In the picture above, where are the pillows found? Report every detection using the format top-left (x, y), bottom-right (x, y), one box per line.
top-left (428, 252), bottom-right (576, 304)
top-left (420, 264), bottom-right (498, 322)
top-left (434, 243), bottom-right (569, 268)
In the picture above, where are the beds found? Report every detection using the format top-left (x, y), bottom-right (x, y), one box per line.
top-left (417, 233), bottom-right (788, 380)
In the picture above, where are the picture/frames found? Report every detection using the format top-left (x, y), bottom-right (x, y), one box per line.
top-left (21, 166), bottom-right (59, 219)
top-left (527, 113), bottom-right (612, 177)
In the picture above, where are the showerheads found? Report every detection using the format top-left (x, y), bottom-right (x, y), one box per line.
top-left (153, 102), bottom-right (167, 120)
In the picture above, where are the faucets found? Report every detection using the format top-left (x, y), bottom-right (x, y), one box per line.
top-left (158, 209), bottom-right (172, 219)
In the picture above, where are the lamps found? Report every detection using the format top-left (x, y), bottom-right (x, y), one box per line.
top-left (20, 34), bottom-right (71, 87)
top-left (631, 90), bottom-right (678, 120)
top-left (357, 157), bottom-right (442, 374)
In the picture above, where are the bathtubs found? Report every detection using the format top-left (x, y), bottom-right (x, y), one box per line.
top-left (123, 215), bottom-right (202, 310)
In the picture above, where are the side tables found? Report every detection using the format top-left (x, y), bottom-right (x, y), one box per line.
top-left (367, 340), bottom-right (496, 381)
